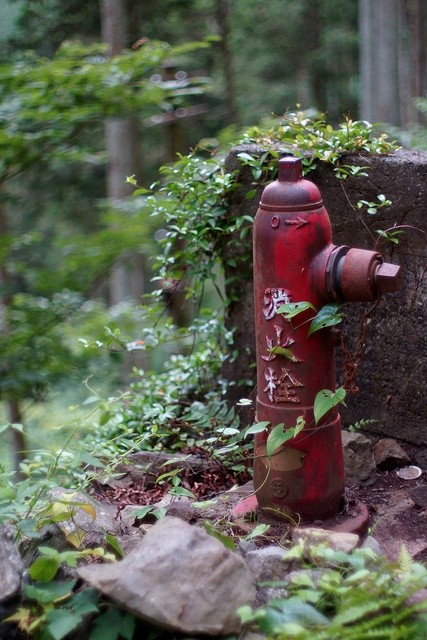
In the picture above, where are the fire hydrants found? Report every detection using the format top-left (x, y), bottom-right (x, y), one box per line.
top-left (232, 156), bottom-right (403, 547)
